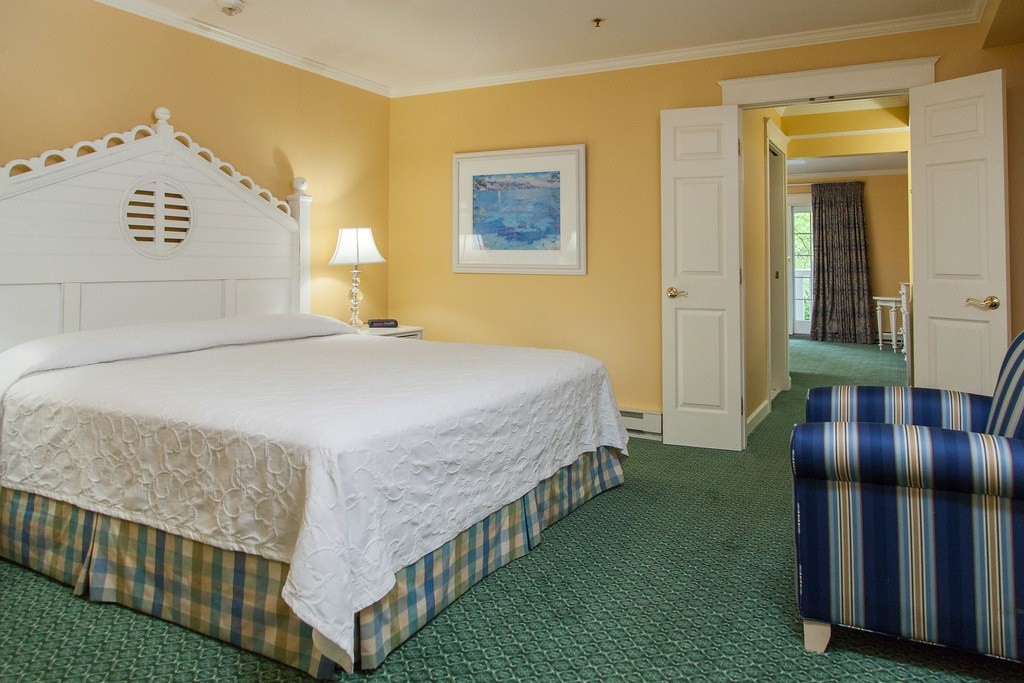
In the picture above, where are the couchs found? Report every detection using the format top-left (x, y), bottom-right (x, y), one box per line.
top-left (787, 332), bottom-right (1024, 673)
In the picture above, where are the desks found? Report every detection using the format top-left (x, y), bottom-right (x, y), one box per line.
top-left (872, 296), bottom-right (902, 354)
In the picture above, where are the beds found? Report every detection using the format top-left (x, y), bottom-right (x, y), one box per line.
top-left (0, 105), bottom-right (630, 682)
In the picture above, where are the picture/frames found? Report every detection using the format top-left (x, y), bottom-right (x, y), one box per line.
top-left (452, 144), bottom-right (587, 276)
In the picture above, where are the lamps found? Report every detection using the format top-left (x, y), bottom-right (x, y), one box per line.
top-left (328, 226), bottom-right (386, 331)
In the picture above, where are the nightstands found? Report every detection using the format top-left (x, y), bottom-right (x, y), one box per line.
top-left (364, 325), bottom-right (424, 341)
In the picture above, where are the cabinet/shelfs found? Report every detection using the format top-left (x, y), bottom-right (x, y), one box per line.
top-left (900, 283), bottom-right (911, 362)
